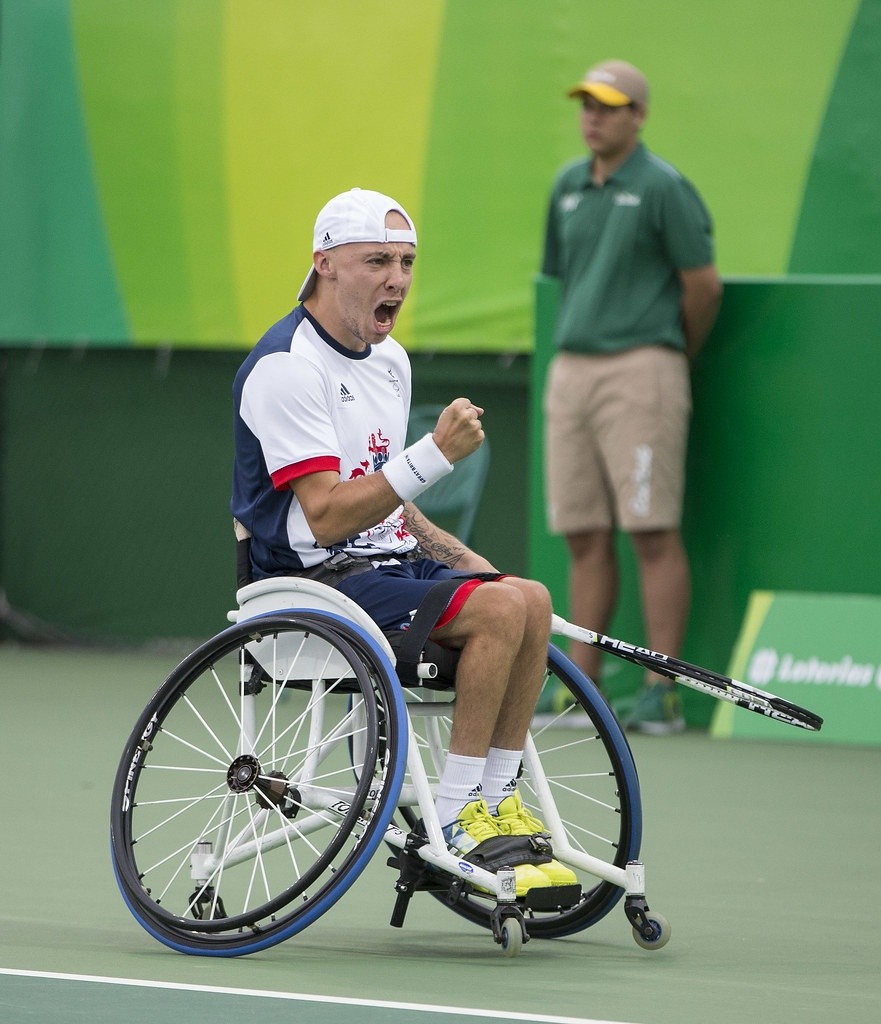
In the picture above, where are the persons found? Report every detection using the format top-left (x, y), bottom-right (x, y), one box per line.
top-left (533, 58), bottom-right (718, 734)
top-left (230, 189), bottom-right (582, 903)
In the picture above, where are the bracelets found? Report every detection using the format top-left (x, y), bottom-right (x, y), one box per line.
top-left (382, 432), bottom-right (453, 504)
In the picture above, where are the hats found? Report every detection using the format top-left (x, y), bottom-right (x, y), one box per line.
top-left (568, 58), bottom-right (649, 112)
top-left (296, 188), bottom-right (418, 302)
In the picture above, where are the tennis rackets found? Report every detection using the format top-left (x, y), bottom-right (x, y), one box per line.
top-left (547, 611), bottom-right (828, 735)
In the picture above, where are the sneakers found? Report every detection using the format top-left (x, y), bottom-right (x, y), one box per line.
top-left (638, 682), bottom-right (685, 735)
top-left (412, 793), bottom-right (553, 903)
top-left (491, 790), bottom-right (577, 887)
top-left (530, 686), bottom-right (617, 729)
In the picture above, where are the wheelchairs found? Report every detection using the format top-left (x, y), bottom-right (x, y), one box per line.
top-left (105, 516), bottom-right (671, 960)
